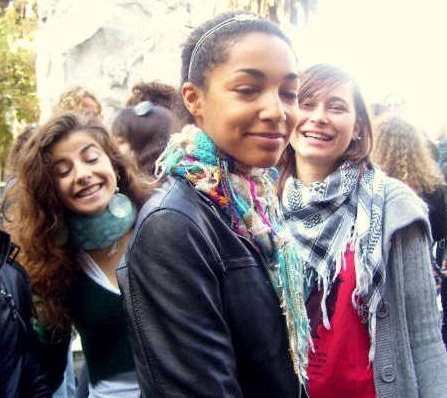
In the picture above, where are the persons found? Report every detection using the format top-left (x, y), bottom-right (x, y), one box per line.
top-left (2, 114), bottom-right (162, 398)
top-left (116, 11), bottom-right (311, 398)
top-left (369, 113), bottom-right (447, 241)
top-left (276, 63), bottom-right (447, 398)
